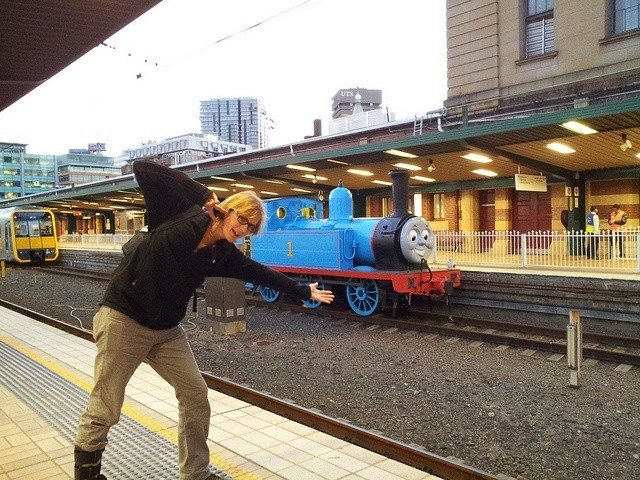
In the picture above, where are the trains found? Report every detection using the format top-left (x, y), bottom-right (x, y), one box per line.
top-left (0, 207), bottom-right (58, 267)
top-left (232, 170), bottom-right (460, 316)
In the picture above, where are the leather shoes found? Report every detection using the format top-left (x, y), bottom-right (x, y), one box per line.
top-left (205, 473), bottom-right (230, 480)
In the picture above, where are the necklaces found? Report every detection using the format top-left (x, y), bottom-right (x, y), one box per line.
top-left (207, 221), bottom-right (217, 264)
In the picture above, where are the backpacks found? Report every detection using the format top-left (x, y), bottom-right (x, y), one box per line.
top-left (121, 204), bottom-right (218, 265)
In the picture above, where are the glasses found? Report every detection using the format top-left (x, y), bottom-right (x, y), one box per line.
top-left (233, 209), bottom-right (257, 233)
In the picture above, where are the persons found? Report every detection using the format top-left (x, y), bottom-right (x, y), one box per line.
top-left (608, 204), bottom-right (627, 259)
top-left (587, 206), bottom-right (600, 259)
top-left (74, 159), bottom-right (335, 480)
